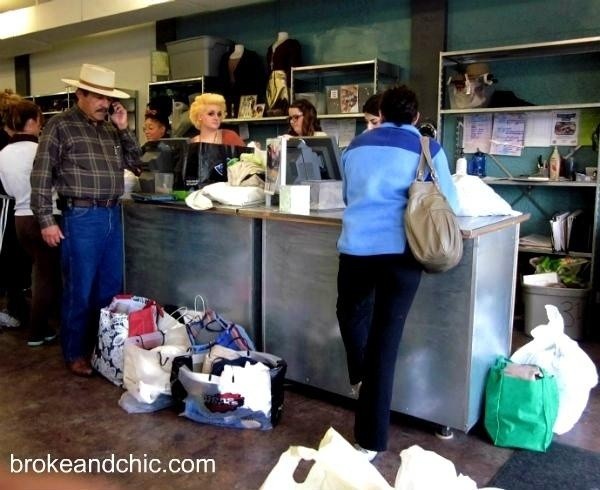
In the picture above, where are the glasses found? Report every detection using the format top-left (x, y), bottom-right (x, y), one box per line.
top-left (286, 115), bottom-right (303, 122)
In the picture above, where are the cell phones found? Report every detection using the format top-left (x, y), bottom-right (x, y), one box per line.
top-left (109, 106), bottom-right (114, 115)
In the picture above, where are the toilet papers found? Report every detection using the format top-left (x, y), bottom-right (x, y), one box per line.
top-left (278, 184), bottom-right (311, 211)
top-left (550, 145), bottom-right (560, 179)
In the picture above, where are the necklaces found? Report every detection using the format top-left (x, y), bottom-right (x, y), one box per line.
top-left (199, 131), bottom-right (219, 143)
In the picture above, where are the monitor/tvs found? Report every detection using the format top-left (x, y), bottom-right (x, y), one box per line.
top-left (139, 138), bottom-right (191, 194)
top-left (285, 137), bottom-right (346, 183)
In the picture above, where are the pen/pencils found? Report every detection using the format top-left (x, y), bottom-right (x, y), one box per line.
top-left (537, 154), bottom-right (549, 168)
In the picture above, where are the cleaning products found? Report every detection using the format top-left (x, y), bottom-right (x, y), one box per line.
top-left (469, 148), bottom-right (486, 176)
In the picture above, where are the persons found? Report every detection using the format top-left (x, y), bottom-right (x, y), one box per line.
top-left (0, 63), bottom-right (143, 376)
top-left (143, 110), bottom-right (173, 143)
top-left (363, 93), bottom-right (382, 130)
top-left (266, 32), bottom-right (303, 116)
top-left (286, 98), bottom-right (327, 137)
top-left (216, 44), bottom-right (266, 119)
top-left (189, 93), bottom-right (246, 148)
top-left (335, 85), bottom-right (458, 464)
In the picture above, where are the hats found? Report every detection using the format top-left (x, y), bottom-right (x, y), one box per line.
top-left (60, 63), bottom-right (130, 99)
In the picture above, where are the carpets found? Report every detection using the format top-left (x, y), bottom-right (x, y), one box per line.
top-left (484, 441), bottom-right (600, 490)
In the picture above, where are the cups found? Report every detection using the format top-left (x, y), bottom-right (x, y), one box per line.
top-left (538, 167), bottom-right (549, 178)
top-left (565, 157), bottom-right (576, 181)
top-left (154, 173), bottom-right (174, 194)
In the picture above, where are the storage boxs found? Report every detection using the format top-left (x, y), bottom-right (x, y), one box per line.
top-left (165, 34), bottom-right (233, 82)
top-left (520, 279), bottom-right (592, 342)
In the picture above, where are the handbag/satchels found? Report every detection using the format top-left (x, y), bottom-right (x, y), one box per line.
top-left (89, 293), bottom-right (289, 433)
top-left (481, 355), bottom-right (560, 455)
top-left (404, 136), bottom-right (464, 273)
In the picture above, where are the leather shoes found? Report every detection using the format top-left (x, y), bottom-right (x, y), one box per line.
top-left (70, 356), bottom-right (92, 376)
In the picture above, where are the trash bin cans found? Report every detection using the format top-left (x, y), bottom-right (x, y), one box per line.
top-left (522, 284), bottom-right (588, 341)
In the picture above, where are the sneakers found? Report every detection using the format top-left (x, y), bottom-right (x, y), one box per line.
top-left (352, 381), bottom-right (362, 400)
top-left (0, 308), bottom-right (22, 329)
top-left (353, 442), bottom-right (378, 462)
top-left (27, 333), bottom-right (57, 346)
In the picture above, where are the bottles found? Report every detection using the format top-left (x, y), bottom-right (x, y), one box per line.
top-left (125, 331), bottom-right (165, 350)
top-left (472, 148), bottom-right (486, 177)
top-left (549, 146), bottom-right (561, 182)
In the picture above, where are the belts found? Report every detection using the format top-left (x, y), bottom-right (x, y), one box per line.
top-left (73, 198), bottom-right (120, 208)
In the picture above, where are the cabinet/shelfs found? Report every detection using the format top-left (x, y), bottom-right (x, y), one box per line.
top-left (287, 58), bottom-right (404, 150)
top-left (435, 36), bottom-right (600, 296)
top-left (148, 76), bottom-right (218, 142)
top-left (22, 88), bottom-right (140, 144)
